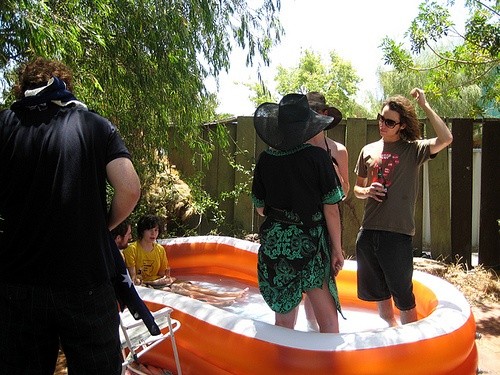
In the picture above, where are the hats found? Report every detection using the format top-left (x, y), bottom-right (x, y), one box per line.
top-left (253, 92), bottom-right (334, 151)
top-left (306, 92), bottom-right (342, 131)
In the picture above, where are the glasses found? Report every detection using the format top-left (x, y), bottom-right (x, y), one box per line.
top-left (377, 113), bottom-right (400, 129)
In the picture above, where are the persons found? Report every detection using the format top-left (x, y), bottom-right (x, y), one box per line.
top-left (304, 91), bottom-right (350, 321)
top-left (353, 88), bottom-right (453, 324)
top-left (0, 58), bottom-right (141, 375)
top-left (112, 214), bottom-right (249, 308)
top-left (250, 94), bottom-right (345, 333)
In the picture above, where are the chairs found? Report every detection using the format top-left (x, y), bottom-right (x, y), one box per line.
top-left (119, 307), bottom-right (182, 375)
